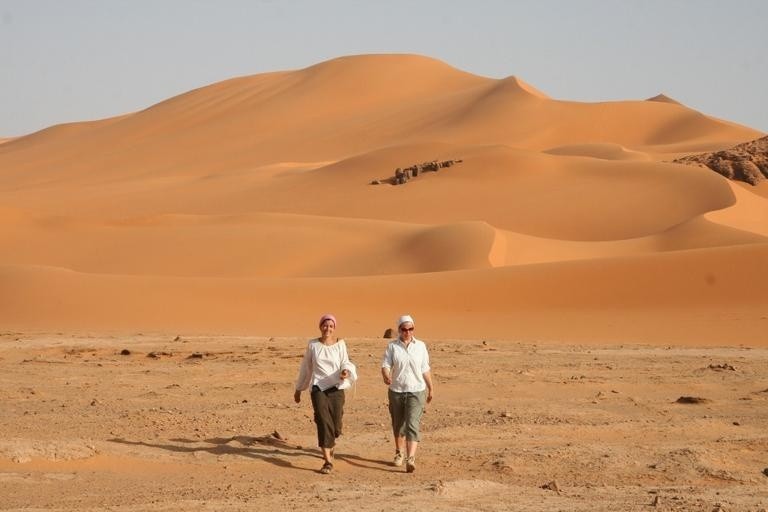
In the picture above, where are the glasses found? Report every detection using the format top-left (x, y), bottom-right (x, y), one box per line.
top-left (400, 327), bottom-right (414, 332)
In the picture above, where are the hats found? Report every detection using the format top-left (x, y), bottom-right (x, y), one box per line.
top-left (319, 315), bottom-right (336, 331)
top-left (398, 315), bottom-right (414, 332)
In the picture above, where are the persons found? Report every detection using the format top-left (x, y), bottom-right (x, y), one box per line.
top-left (292, 314), bottom-right (359, 475)
top-left (380, 314), bottom-right (434, 474)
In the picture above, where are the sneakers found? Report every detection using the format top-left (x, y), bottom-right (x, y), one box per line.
top-left (406, 455), bottom-right (416, 472)
top-left (320, 462), bottom-right (333, 474)
top-left (391, 448), bottom-right (404, 467)
